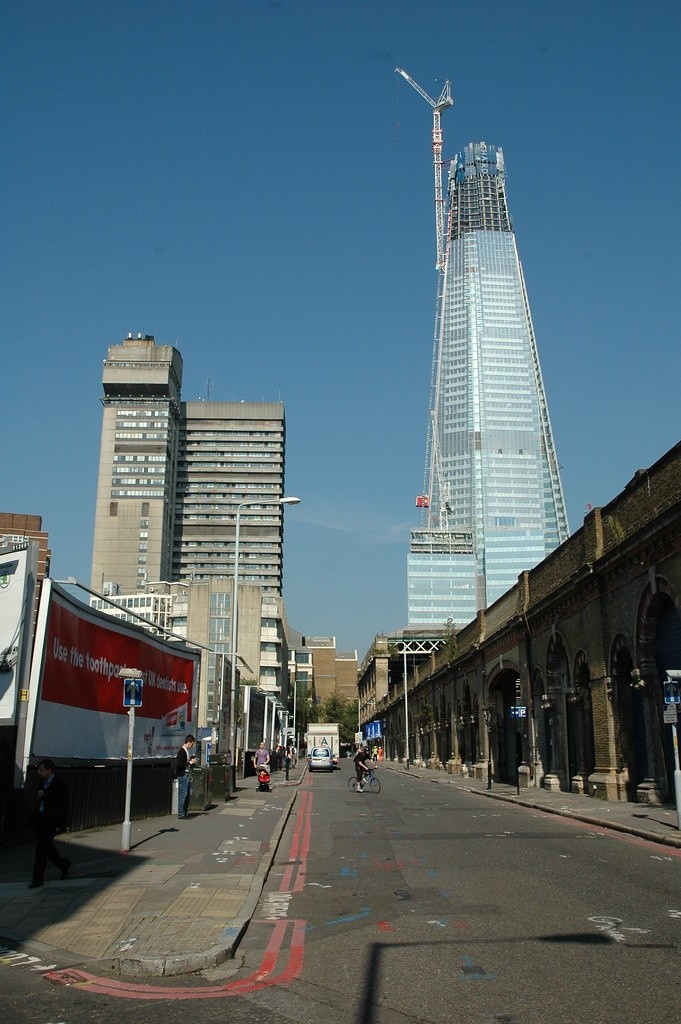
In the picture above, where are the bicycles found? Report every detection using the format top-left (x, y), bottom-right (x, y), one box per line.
top-left (348, 763), bottom-right (381, 794)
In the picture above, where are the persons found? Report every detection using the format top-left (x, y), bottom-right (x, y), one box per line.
top-left (176, 735), bottom-right (197, 820)
top-left (302, 748), bottom-right (305, 757)
top-left (372, 746), bottom-right (383, 762)
top-left (28, 759), bottom-right (71, 889)
top-left (254, 742), bottom-right (270, 774)
top-left (289, 744), bottom-right (297, 767)
top-left (355, 747), bottom-right (378, 792)
top-left (258, 771), bottom-right (271, 788)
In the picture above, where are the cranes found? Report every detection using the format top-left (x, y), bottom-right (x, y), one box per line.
top-left (393, 65), bottom-right (457, 552)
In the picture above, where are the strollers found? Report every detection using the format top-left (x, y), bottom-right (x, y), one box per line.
top-left (255, 762), bottom-right (272, 792)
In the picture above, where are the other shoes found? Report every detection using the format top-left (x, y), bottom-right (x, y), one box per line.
top-left (356, 786), bottom-right (363, 792)
top-left (178, 815), bottom-right (191, 819)
top-left (363, 778), bottom-right (368, 783)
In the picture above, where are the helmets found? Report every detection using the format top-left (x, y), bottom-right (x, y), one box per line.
top-left (364, 747), bottom-right (370, 754)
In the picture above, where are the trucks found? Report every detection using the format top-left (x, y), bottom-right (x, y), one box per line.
top-left (303, 722), bottom-right (340, 765)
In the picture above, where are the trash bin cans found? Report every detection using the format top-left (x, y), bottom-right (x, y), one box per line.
top-left (209, 753), bottom-right (234, 801)
top-left (188, 767), bottom-right (212, 810)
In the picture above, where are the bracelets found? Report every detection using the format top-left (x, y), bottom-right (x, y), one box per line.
top-left (374, 765), bottom-right (376, 766)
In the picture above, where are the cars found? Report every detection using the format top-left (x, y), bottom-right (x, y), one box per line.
top-left (305, 746), bottom-right (336, 773)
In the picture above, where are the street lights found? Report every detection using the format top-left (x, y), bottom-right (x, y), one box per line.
top-left (377, 640), bottom-right (410, 770)
top-left (229, 496), bottom-right (304, 801)
top-left (346, 696), bottom-right (361, 750)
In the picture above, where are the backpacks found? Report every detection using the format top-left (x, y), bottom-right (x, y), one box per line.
top-left (353, 752), bottom-right (365, 763)
